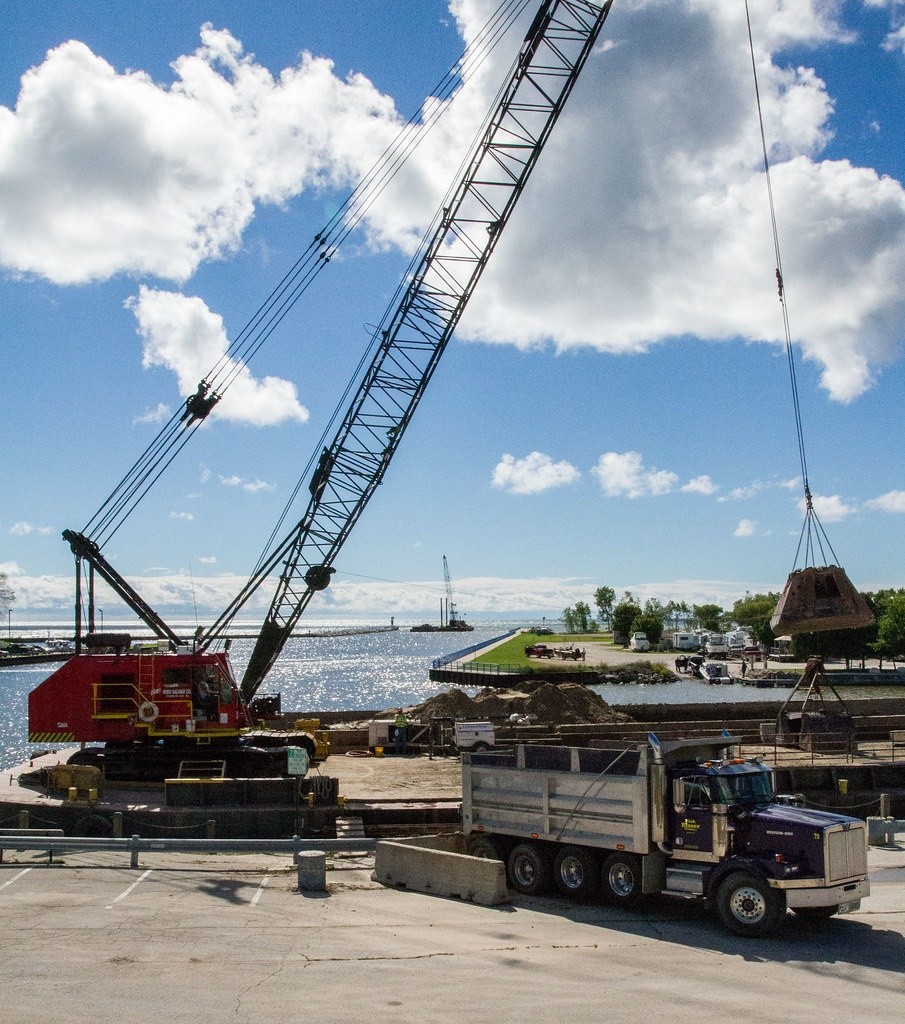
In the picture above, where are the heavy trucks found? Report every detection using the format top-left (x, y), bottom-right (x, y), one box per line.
top-left (456, 737), bottom-right (873, 941)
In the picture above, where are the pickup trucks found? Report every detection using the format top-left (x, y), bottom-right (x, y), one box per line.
top-left (688, 655), bottom-right (707, 674)
top-left (0, 643), bottom-right (32, 655)
top-left (729, 643), bottom-right (743, 657)
top-left (807, 655), bottom-right (824, 667)
top-left (524, 644), bottom-right (553, 659)
top-left (743, 647), bottom-right (762, 662)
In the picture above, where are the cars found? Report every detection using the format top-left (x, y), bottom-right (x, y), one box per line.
top-left (31, 647), bottom-right (40, 655)
top-left (0, 649), bottom-right (9, 658)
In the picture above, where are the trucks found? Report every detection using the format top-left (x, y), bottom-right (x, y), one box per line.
top-left (628, 632), bottom-right (651, 652)
top-left (673, 633), bottom-right (700, 652)
top-left (707, 634), bottom-right (727, 660)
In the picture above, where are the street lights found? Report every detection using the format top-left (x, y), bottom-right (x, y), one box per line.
top-left (99, 609), bottom-right (104, 634)
top-left (8, 609), bottom-right (13, 641)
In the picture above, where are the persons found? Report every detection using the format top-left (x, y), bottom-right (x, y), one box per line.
top-left (198, 672), bottom-right (218, 719)
top-left (570, 643), bottom-right (574, 650)
top-left (741, 660), bottom-right (749, 678)
top-left (582, 648), bottom-right (586, 661)
top-left (395, 707), bottom-right (407, 754)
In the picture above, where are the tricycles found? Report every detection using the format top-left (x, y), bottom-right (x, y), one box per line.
top-left (674, 657), bottom-right (689, 672)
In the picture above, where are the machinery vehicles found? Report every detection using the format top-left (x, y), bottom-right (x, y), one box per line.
top-left (27, 0), bottom-right (877, 798)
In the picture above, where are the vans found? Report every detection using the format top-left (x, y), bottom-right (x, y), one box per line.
top-left (35, 645), bottom-right (51, 654)
